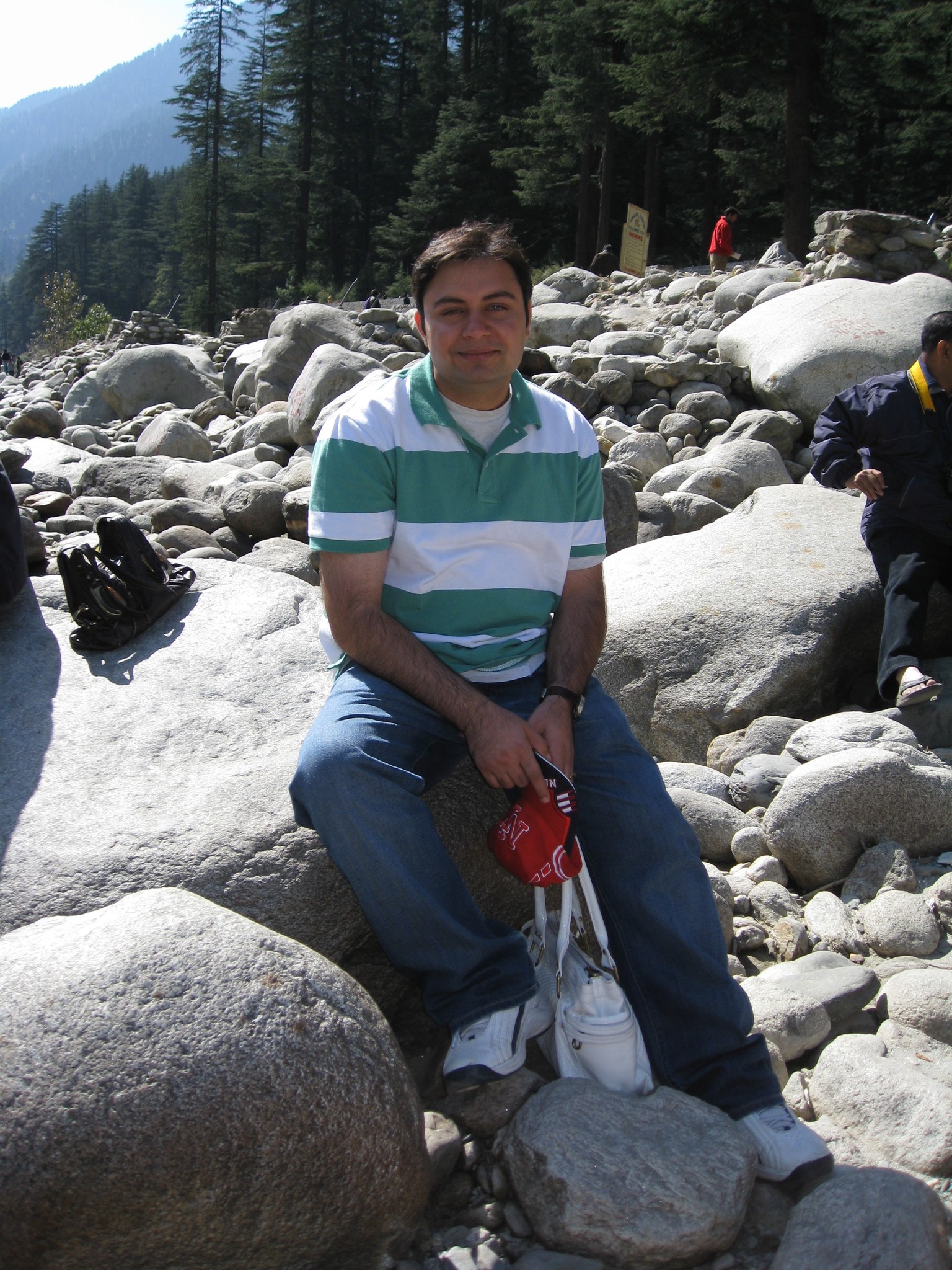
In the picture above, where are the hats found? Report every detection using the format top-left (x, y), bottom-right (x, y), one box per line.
top-left (372, 289), bottom-right (379, 295)
top-left (489, 747), bottom-right (584, 889)
top-left (725, 207), bottom-right (738, 216)
top-left (603, 244), bottom-right (612, 250)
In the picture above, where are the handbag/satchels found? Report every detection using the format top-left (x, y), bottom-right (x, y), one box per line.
top-left (56, 512), bottom-right (197, 651)
top-left (519, 834), bottom-right (657, 1100)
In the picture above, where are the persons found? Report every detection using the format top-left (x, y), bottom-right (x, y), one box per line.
top-left (708, 207), bottom-right (739, 274)
top-left (364, 289), bottom-right (381, 308)
top-left (0, 349), bottom-right (23, 378)
top-left (590, 244), bottom-right (620, 277)
top-left (809, 310), bottom-right (952, 706)
top-left (289, 220), bottom-right (835, 1183)
top-left (0, 462), bottom-right (29, 604)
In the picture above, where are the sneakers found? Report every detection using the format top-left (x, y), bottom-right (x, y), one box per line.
top-left (737, 1103), bottom-right (835, 1187)
top-left (439, 990), bottom-right (556, 1094)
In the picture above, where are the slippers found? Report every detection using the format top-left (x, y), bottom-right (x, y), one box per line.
top-left (892, 674), bottom-right (944, 708)
